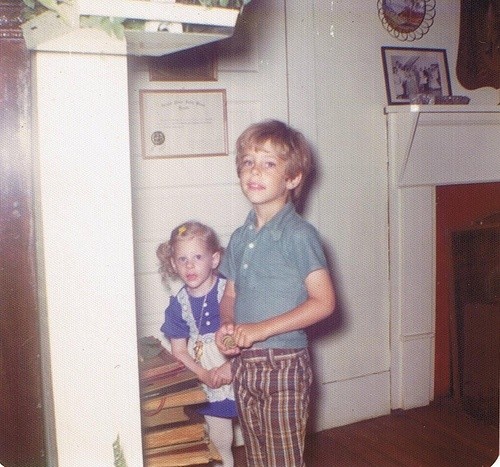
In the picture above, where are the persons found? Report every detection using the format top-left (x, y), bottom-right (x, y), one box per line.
top-left (216, 120), bottom-right (336, 467)
top-left (157, 222), bottom-right (237, 467)
top-left (392, 61), bottom-right (441, 99)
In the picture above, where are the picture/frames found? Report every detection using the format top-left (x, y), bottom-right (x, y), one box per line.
top-left (150, 43), bottom-right (217, 81)
top-left (380, 46), bottom-right (452, 105)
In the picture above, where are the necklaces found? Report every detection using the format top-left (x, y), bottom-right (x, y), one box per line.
top-left (187, 275), bottom-right (216, 363)
